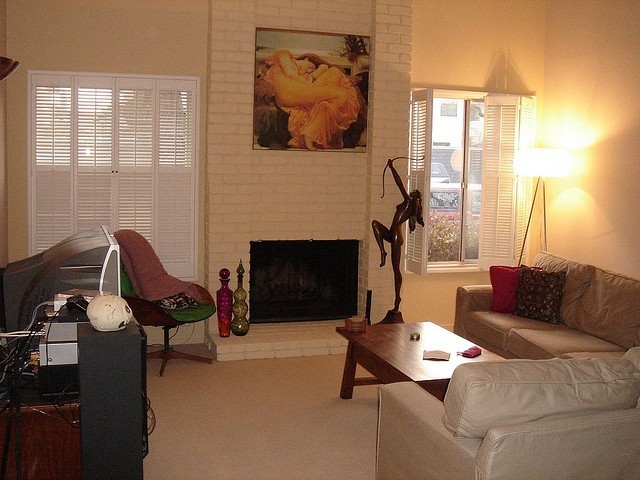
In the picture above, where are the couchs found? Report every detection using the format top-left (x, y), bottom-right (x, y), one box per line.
top-left (453, 251), bottom-right (640, 359)
top-left (375, 346), bottom-right (640, 479)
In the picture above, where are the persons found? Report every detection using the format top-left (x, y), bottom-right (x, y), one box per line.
top-left (260, 50), bottom-right (361, 151)
top-left (371, 159), bottom-right (424, 311)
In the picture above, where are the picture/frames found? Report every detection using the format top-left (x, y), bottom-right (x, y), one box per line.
top-left (252, 27), bottom-right (371, 154)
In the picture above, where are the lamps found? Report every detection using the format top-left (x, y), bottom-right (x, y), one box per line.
top-left (511, 145), bottom-right (560, 268)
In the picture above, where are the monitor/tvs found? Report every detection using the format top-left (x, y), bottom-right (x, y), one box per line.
top-left (0, 224), bottom-right (121, 343)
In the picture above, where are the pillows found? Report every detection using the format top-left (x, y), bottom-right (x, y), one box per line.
top-left (510, 261), bottom-right (568, 326)
top-left (488, 262), bottom-right (542, 315)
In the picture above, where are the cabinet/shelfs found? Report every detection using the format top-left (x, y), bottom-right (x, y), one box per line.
top-left (0, 399), bottom-right (83, 479)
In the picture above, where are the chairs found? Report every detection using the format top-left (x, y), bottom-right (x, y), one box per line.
top-left (113, 229), bottom-right (216, 376)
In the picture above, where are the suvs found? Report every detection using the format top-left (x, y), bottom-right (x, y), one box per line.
top-left (429, 182), bottom-right (481, 221)
top-left (429, 162), bottom-right (449, 185)
